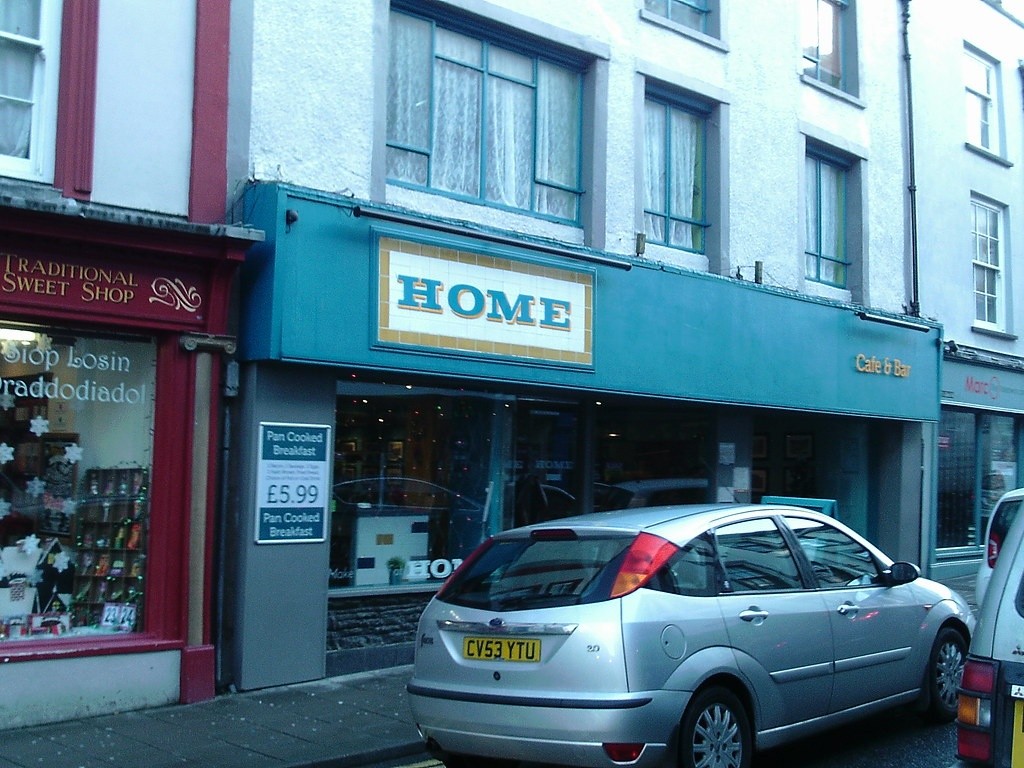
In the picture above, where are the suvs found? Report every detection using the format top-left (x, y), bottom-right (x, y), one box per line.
top-left (975, 487), bottom-right (1024, 610)
top-left (954, 500), bottom-right (1024, 768)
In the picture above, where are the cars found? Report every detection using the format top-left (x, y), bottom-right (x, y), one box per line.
top-left (403, 500), bottom-right (974, 768)
top-left (332, 475), bottom-right (709, 589)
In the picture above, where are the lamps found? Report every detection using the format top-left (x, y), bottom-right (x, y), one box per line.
top-left (352, 204), bottom-right (634, 271)
top-left (944, 339), bottom-right (959, 353)
top-left (854, 310), bottom-right (931, 334)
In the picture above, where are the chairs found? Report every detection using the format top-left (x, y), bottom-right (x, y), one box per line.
top-left (718, 539), bottom-right (792, 594)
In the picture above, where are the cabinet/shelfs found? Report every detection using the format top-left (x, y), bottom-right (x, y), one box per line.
top-left (71, 467), bottom-right (149, 634)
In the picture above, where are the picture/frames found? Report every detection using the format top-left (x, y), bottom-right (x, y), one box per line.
top-left (783, 431), bottom-right (817, 460)
top-left (752, 432), bottom-right (771, 462)
top-left (752, 466), bottom-right (770, 494)
top-left (782, 466), bottom-right (817, 495)
top-left (384, 439), bottom-right (406, 463)
top-left (340, 439), bottom-right (359, 453)
